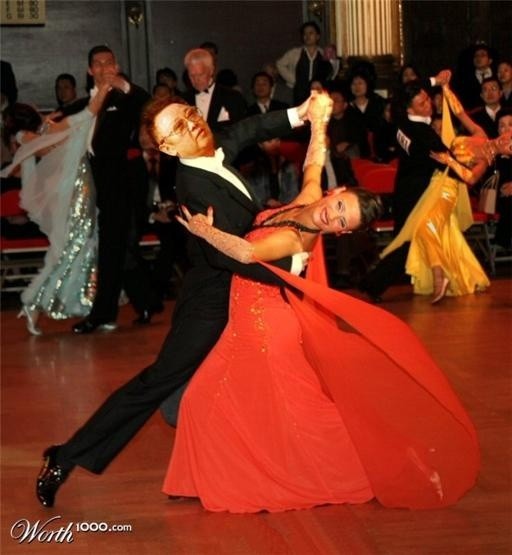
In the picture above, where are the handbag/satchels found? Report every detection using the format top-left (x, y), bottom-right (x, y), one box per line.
top-left (478, 170), bottom-right (500, 216)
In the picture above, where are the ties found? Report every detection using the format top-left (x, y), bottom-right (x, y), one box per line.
top-left (264, 104), bottom-right (269, 114)
top-left (480, 71), bottom-right (487, 82)
top-left (145, 156), bottom-right (159, 212)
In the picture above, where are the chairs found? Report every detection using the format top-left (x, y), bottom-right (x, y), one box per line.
top-left (0, 142), bottom-right (512, 306)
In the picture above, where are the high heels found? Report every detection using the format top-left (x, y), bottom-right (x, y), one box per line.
top-left (430, 276), bottom-right (454, 306)
top-left (97, 322), bottom-right (119, 331)
top-left (16, 304), bottom-right (44, 336)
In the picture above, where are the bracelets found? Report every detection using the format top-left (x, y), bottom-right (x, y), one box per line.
top-left (308, 117), bottom-right (323, 127)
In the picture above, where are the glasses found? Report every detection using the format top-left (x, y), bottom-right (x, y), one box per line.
top-left (160, 106), bottom-right (204, 145)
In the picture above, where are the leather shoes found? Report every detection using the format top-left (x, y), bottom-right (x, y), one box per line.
top-left (35, 445), bottom-right (69, 509)
top-left (71, 313), bottom-right (101, 335)
top-left (132, 301), bottom-right (166, 326)
top-left (358, 282), bottom-right (382, 304)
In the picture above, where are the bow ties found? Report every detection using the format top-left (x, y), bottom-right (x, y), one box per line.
top-left (194, 87), bottom-right (210, 95)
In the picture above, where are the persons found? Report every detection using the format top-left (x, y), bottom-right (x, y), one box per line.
top-left (174, 96), bottom-right (481, 512)
top-left (35, 90), bottom-right (333, 509)
